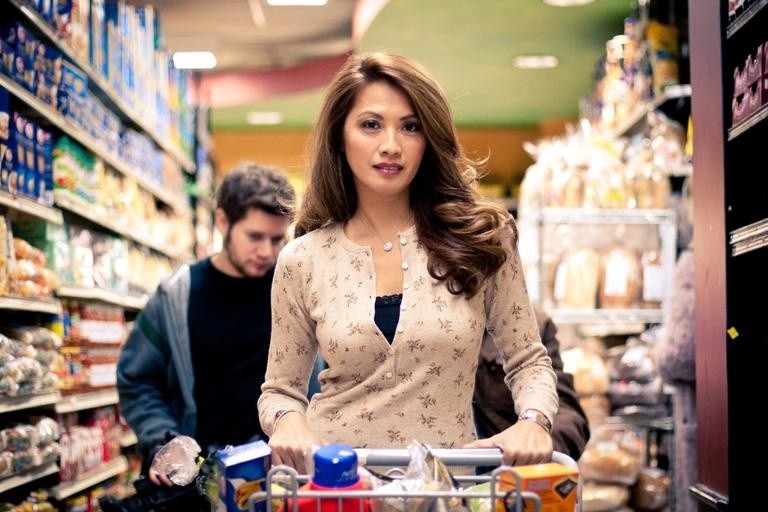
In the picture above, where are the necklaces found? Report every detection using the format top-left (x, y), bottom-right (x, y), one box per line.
top-left (357, 212), bottom-right (412, 251)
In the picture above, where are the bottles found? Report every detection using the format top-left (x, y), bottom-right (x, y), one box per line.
top-left (277, 444), bottom-right (384, 512)
top-left (97, 408), bottom-right (122, 466)
top-left (81, 300), bottom-right (126, 392)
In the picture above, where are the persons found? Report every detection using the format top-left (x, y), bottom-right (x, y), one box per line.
top-left (117, 161), bottom-right (323, 487)
top-left (256, 50), bottom-right (560, 509)
top-left (471, 304), bottom-right (590, 463)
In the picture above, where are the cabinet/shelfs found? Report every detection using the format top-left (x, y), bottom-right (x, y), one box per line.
top-left (689, 0), bottom-right (768, 512)
top-left (584, 4), bottom-right (688, 229)
top-left (2, 1), bottom-right (228, 510)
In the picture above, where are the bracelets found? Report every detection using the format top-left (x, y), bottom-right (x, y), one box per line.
top-left (517, 411), bottom-right (551, 433)
top-left (273, 410), bottom-right (292, 433)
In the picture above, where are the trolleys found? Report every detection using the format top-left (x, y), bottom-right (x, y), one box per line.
top-left (249, 447), bottom-right (583, 512)
